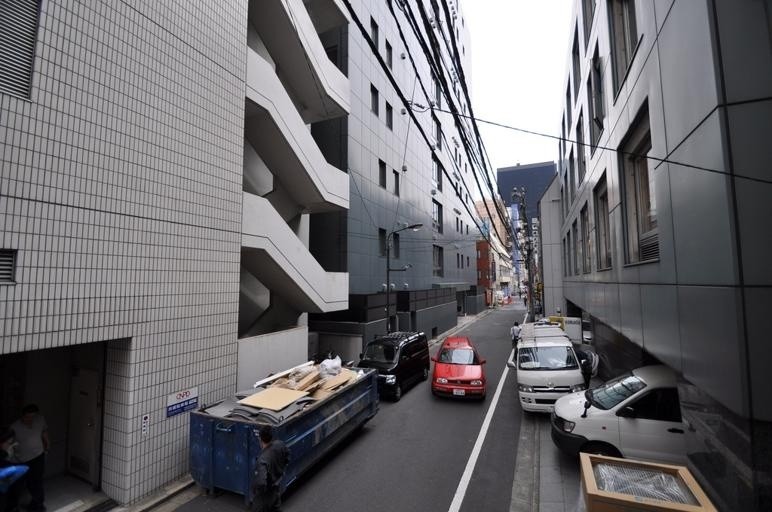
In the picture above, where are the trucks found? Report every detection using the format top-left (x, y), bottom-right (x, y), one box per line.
top-left (507, 321), bottom-right (587, 415)
top-left (539, 317), bottom-right (583, 345)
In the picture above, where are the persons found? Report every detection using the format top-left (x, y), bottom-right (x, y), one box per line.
top-left (1, 427), bottom-right (16, 468)
top-left (10, 403), bottom-right (49, 511)
top-left (576, 352), bottom-right (592, 389)
top-left (523, 296), bottom-right (527, 306)
top-left (250, 428), bottom-right (287, 511)
top-left (510, 322), bottom-right (521, 360)
top-left (320, 352), bottom-right (342, 368)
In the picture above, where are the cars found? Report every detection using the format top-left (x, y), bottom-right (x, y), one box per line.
top-left (430, 334), bottom-right (488, 402)
top-left (582, 320), bottom-right (592, 343)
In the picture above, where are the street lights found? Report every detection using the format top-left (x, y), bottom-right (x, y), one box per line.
top-left (385, 222), bottom-right (424, 329)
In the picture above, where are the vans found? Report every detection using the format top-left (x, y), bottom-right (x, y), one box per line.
top-left (551, 365), bottom-right (688, 467)
top-left (358, 330), bottom-right (432, 401)
